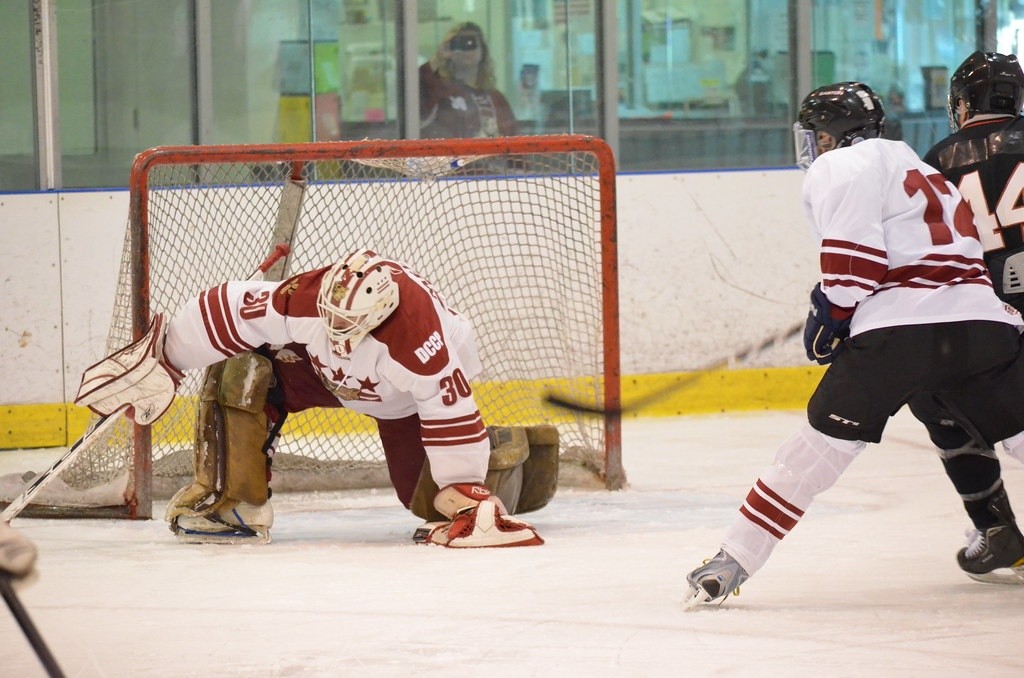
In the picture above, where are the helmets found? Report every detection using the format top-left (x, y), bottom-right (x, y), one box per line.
top-left (949, 51), bottom-right (1024, 118)
top-left (316, 248), bottom-right (399, 357)
top-left (799, 82), bottom-right (886, 150)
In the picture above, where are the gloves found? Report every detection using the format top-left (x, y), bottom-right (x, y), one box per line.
top-left (803, 282), bottom-right (858, 365)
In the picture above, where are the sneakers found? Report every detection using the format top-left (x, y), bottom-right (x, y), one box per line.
top-left (957, 524), bottom-right (1024, 585)
top-left (177, 498), bottom-right (274, 548)
top-left (680, 548), bottom-right (749, 612)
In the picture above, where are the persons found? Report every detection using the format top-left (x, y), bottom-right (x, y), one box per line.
top-left (688, 82), bottom-right (1024, 618)
top-left (921, 50), bottom-right (1023, 320)
top-left (419, 22), bottom-right (528, 175)
top-left (76, 249), bottom-right (547, 547)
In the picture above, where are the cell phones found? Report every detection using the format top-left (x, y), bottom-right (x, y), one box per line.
top-left (450, 36), bottom-right (477, 51)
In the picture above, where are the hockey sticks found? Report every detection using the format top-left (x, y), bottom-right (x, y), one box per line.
top-left (541, 319), bottom-right (809, 419)
top-left (0, 571), bottom-right (67, 678)
top-left (1, 405), bottom-right (130, 522)
